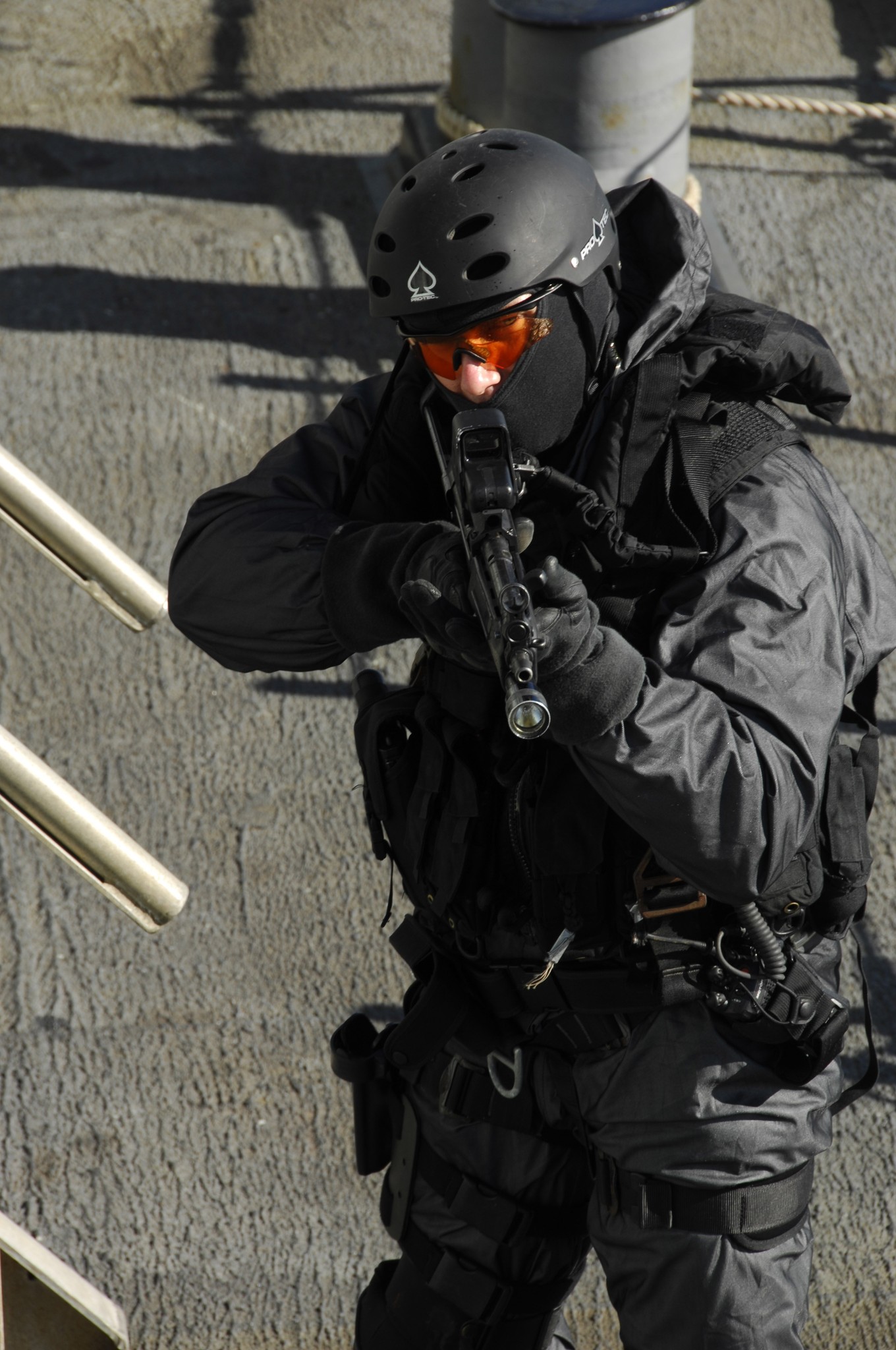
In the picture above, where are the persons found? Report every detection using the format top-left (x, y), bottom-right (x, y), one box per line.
top-left (170, 126), bottom-right (896, 1350)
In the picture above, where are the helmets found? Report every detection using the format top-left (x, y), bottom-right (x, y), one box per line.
top-left (366, 129), bottom-right (619, 325)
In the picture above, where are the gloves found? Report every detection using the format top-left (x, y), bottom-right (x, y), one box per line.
top-left (394, 516), bottom-right (591, 679)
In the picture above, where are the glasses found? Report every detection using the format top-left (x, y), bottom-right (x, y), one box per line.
top-left (416, 314), bottom-right (554, 381)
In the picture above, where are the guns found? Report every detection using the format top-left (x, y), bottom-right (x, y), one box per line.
top-left (417, 380), bottom-right (551, 739)
